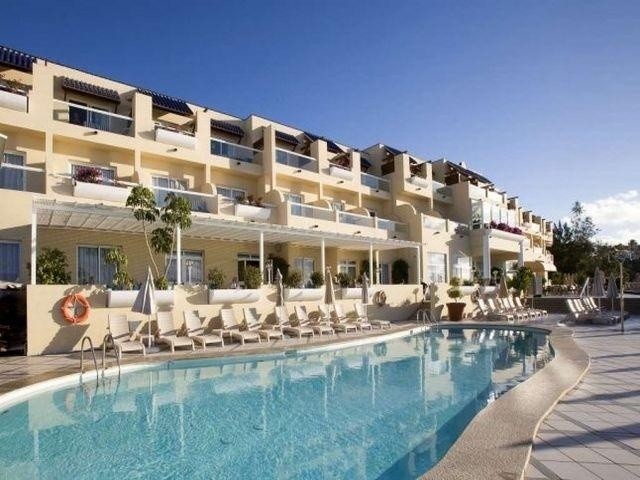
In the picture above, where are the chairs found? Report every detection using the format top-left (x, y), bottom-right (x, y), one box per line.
top-left (473, 297), bottom-right (547, 322)
top-left (564, 297), bottom-right (610, 325)
top-left (107, 303), bottom-right (391, 359)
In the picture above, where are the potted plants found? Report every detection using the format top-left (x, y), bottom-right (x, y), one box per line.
top-left (446, 277), bottom-right (466, 321)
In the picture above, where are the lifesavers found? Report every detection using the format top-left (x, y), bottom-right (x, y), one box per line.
top-left (62, 294), bottom-right (89, 324)
top-left (378, 291), bottom-right (385, 306)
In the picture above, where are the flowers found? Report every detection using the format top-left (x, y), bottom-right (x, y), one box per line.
top-left (491, 221), bottom-right (521, 236)
top-left (73, 166), bottom-right (102, 184)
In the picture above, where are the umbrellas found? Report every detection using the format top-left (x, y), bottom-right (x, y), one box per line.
top-left (323, 267), bottom-right (337, 336)
top-left (590, 266), bottom-right (606, 310)
top-left (130, 265), bottom-right (161, 355)
top-left (137, 370), bottom-right (159, 452)
top-left (273, 268), bottom-right (290, 342)
top-left (498, 273), bottom-right (509, 313)
top-left (606, 272), bottom-right (619, 310)
top-left (360, 272), bottom-right (370, 324)
